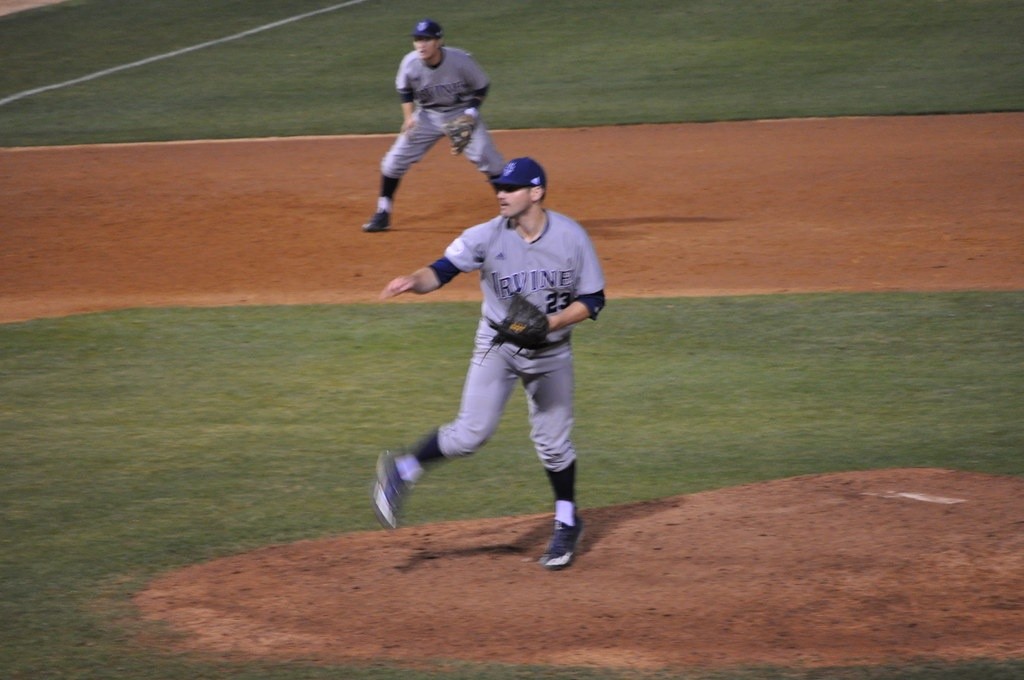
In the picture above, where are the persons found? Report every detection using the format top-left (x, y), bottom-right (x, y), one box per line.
top-left (376, 156), bottom-right (606, 569)
top-left (361, 19), bottom-right (506, 232)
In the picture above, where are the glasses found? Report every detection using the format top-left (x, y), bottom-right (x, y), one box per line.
top-left (495, 185), bottom-right (526, 193)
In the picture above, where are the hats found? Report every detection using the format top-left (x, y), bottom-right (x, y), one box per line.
top-left (409, 19), bottom-right (443, 38)
top-left (484, 157), bottom-right (546, 186)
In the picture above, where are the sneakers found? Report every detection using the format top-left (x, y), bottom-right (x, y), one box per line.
top-left (362, 208), bottom-right (390, 232)
top-left (537, 517), bottom-right (585, 570)
top-left (370, 450), bottom-right (408, 530)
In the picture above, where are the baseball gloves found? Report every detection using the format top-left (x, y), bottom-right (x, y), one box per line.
top-left (481, 293), bottom-right (550, 363)
top-left (441, 114), bottom-right (478, 154)
